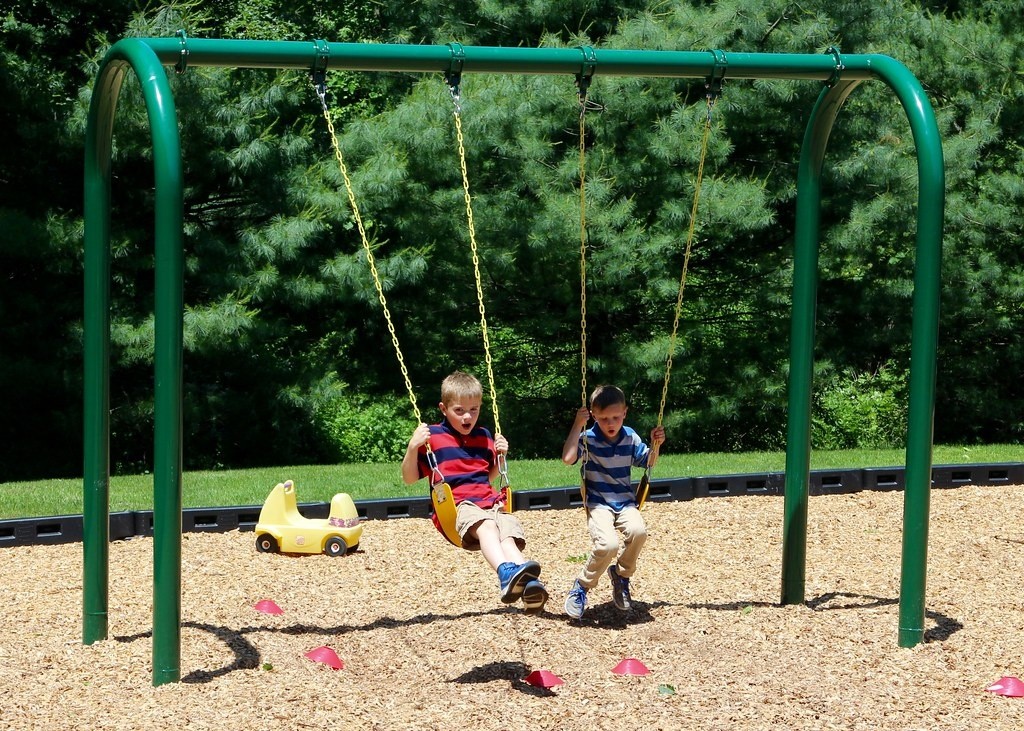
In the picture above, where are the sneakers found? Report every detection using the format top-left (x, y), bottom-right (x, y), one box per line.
top-left (498, 560), bottom-right (541, 603)
top-left (564, 579), bottom-right (588, 622)
top-left (520, 579), bottom-right (548, 613)
top-left (608, 564), bottom-right (635, 610)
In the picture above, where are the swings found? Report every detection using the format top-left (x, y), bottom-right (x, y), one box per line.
top-left (308, 36), bottom-right (515, 552)
top-left (572, 45), bottom-right (729, 521)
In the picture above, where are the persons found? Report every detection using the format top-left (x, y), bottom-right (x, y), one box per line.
top-left (562, 385), bottom-right (666, 619)
top-left (402, 369), bottom-right (548, 614)
